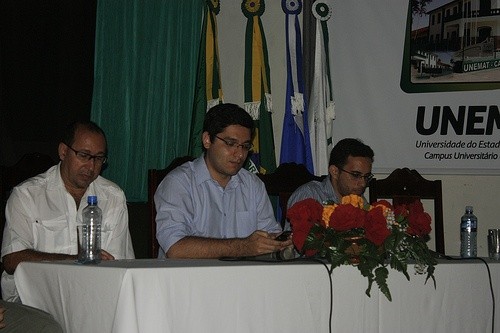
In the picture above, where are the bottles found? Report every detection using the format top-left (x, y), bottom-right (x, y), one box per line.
top-left (81, 195), bottom-right (102, 265)
top-left (460, 206), bottom-right (477, 258)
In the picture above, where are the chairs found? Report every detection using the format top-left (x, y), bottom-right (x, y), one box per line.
top-left (148, 156), bottom-right (197, 259)
top-left (369, 168), bottom-right (445, 256)
top-left (255, 162), bottom-right (328, 228)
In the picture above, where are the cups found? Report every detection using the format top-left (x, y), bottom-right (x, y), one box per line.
top-left (487, 229), bottom-right (500, 262)
top-left (77, 225), bottom-right (101, 263)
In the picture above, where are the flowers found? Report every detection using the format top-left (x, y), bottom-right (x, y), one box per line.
top-left (286, 195), bottom-right (437, 301)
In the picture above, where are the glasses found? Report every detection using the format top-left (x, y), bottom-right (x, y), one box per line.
top-left (339, 169), bottom-right (373, 182)
top-left (64, 143), bottom-right (107, 165)
top-left (213, 134), bottom-right (254, 152)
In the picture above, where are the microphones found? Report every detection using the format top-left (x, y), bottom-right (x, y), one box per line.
top-left (428, 249), bottom-right (445, 258)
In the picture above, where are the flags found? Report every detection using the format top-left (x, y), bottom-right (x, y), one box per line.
top-left (191, 5), bottom-right (223, 159)
top-left (277, 15), bottom-right (314, 223)
top-left (244, 16), bottom-right (279, 220)
top-left (308, 19), bottom-right (333, 177)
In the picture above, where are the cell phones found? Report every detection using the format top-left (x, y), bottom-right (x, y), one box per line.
top-left (274, 231), bottom-right (293, 241)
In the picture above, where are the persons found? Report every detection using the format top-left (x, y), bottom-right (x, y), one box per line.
top-left (0, 120), bottom-right (135, 303)
top-left (284, 138), bottom-right (374, 231)
top-left (0, 300), bottom-right (64, 333)
top-left (154, 102), bottom-right (294, 260)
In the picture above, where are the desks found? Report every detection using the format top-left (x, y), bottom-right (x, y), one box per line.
top-left (14, 257), bottom-right (500, 333)
